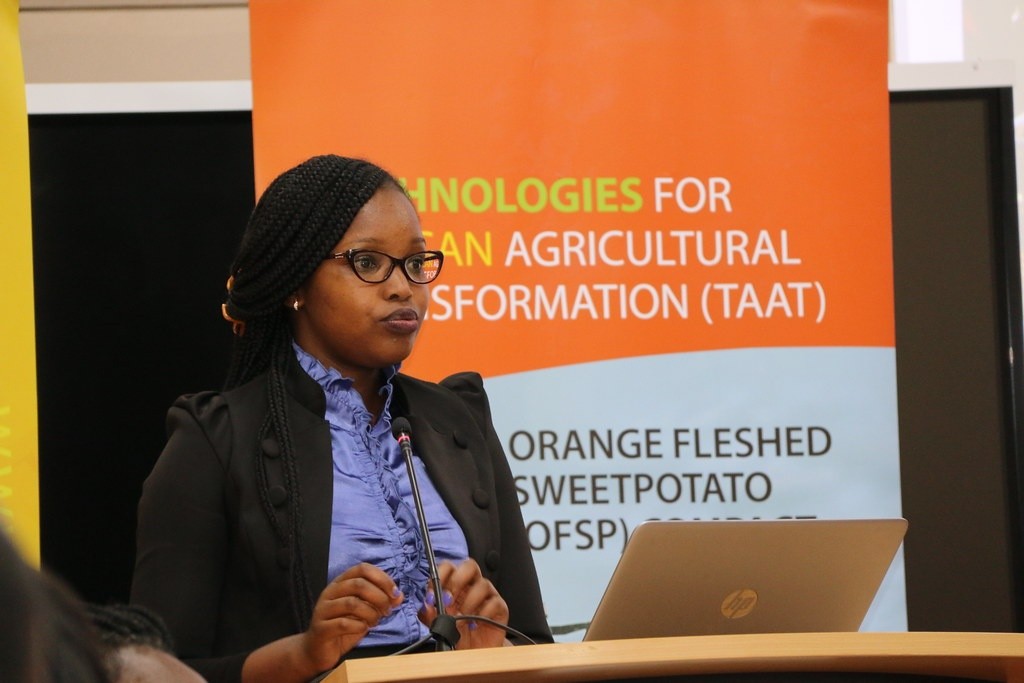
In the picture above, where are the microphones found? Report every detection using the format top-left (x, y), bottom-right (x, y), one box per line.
top-left (391, 417), bottom-right (462, 652)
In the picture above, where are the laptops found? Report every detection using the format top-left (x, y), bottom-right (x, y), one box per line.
top-left (579, 519), bottom-right (909, 633)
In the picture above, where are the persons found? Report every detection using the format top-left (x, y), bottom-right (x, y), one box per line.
top-left (0, 522), bottom-right (207, 683)
top-left (125, 154), bottom-right (557, 683)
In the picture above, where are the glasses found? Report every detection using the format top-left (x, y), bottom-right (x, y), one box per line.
top-left (322, 248), bottom-right (444, 285)
